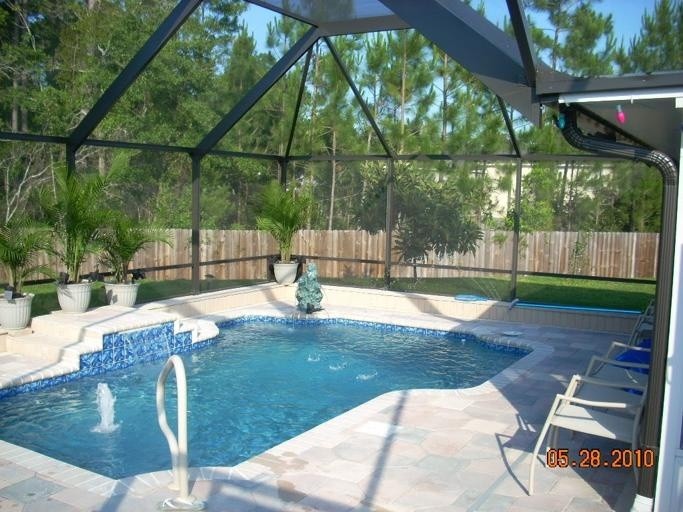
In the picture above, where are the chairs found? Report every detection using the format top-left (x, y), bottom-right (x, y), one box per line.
top-left (528, 299), bottom-right (656, 495)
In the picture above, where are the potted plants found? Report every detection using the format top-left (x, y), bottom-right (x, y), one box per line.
top-left (253, 178), bottom-right (324, 286)
top-left (0, 156), bottom-right (173, 330)
